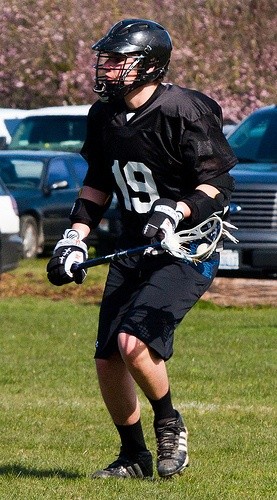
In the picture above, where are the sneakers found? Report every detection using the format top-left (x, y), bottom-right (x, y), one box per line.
top-left (153, 409), bottom-right (191, 479)
top-left (91, 446), bottom-right (154, 480)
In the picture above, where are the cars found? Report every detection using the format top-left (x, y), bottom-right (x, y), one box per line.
top-left (0, 190), bottom-right (21, 277)
top-left (0, 105), bottom-right (96, 151)
top-left (1, 151), bottom-right (109, 258)
top-left (219, 107), bottom-right (277, 277)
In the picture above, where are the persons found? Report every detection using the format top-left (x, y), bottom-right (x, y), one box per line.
top-left (47, 20), bottom-right (239, 479)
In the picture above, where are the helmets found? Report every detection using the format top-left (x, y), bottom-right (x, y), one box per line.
top-left (91, 18), bottom-right (173, 102)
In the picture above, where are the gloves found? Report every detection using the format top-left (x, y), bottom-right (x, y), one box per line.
top-left (46, 229), bottom-right (89, 286)
top-left (139, 198), bottom-right (186, 259)
